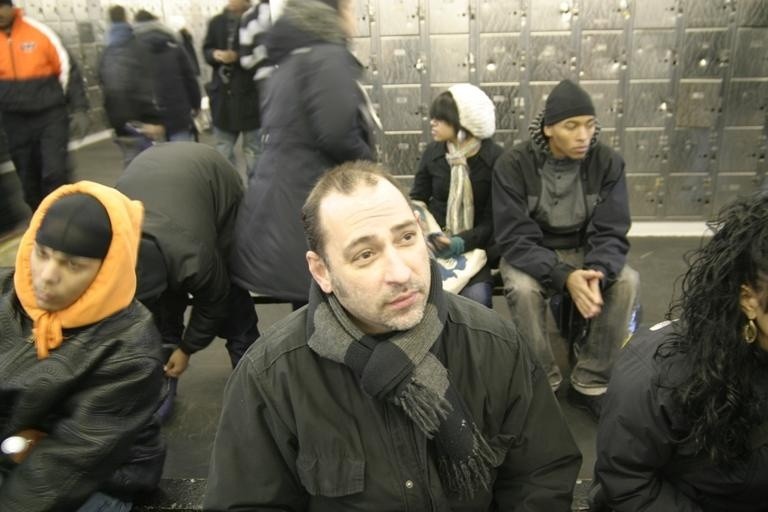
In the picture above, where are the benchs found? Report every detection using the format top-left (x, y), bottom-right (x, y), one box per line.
top-left (189, 264), bottom-right (590, 364)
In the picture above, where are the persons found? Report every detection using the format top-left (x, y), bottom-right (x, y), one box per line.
top-left (200, 156), bottom-right (586, 512)
top-left (228, 0), bottom-right (385, 310)
top-left (98, 0), bottom-right (270, 175)
top-left (492, 76), bottom-right (643, 401)
top-left (588, 194), bottom-right (768, 512)
top-left (0, 1), bottom-right (93, 213)
top-left (114, 141), bottom-right (261, 395)
top-left (407, 81), bottom-right (506, 308)
top-left (0, 179), bottom-right (169, 512)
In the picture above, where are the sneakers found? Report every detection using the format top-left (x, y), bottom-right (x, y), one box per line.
top-left (554, 379), bottom-right (608, 424)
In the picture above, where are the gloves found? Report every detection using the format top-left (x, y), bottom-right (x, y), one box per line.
top-left (67, 109), bottom-right (96, 140)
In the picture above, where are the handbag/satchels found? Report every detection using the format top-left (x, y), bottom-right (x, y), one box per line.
top-left (406, 199), bottom-right (487, 296)
top-left (548, 292), bottom-right (642, 368)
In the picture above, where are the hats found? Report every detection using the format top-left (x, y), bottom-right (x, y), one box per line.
top-left (34, 192), bottom-right (113, 260)
top-left (542, 77), bottom-right (597, 125)
top-left (446, 83), bottom-right (496, 141)
